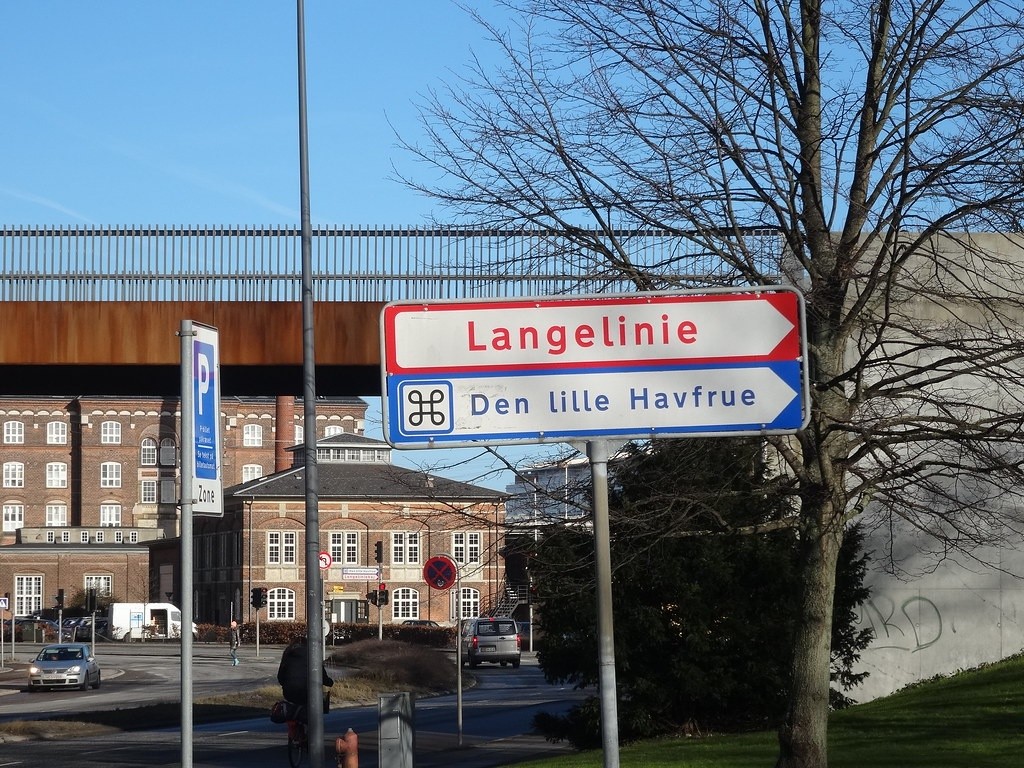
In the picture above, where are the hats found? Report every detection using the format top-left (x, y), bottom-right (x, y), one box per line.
top-left (231, 621), bottom-right (236, 627)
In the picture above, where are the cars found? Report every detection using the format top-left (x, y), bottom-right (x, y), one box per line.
top-left (0, 617), bottom-right (109, 640)
top-left (400, 620), bottom-right (439, 626)
top-left (27, 643), bottom-right (101, 690)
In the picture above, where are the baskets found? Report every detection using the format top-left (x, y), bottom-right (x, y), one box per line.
top-left (323, 691), bottom-right (330, 714)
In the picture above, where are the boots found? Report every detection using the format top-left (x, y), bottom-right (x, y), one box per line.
top-left (232, 658), bottom-right (239, 667)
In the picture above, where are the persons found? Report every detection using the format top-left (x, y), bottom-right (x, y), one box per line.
top-left (229, 621), bottom-right (241, 666)
top-left (277, 619), bottom-right (334, 768)
top-left (45, 654), bottom-right (59, 660)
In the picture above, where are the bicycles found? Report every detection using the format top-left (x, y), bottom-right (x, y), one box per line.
top-left (272, 682), bottom-right (332, 768)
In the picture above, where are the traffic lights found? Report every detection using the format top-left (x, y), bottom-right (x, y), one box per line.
top-left (386, 589), bottom-right (389, 604)
top-left (250, 588), bottom-right (255, 609)
top-left (374, 541), bottom-right (383, 564)
top-left (374, 540), bottom-right (383, 563)
top-left (260, 587), bottom-right (268, 608)
top-left (379, 582), bottom-right (387, 605)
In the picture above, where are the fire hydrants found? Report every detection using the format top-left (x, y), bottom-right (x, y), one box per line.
top-left (335, 727), bottom-right (360, 768)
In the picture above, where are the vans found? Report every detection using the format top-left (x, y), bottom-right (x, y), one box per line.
top-left (108, 602), bottom-right (201, 642)
top-left (457, 616), bottom-right (522, 668)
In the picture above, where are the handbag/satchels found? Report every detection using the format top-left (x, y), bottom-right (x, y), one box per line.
top-left (271, 700), bottom-right (287, 724)
